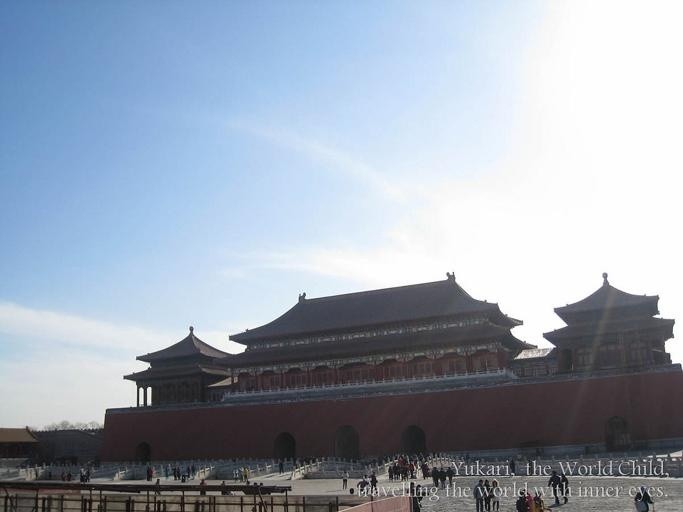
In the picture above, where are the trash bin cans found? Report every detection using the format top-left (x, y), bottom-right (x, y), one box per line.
top-left (80, 475), bottom-right (87, 482)
top-left (182, 475), bottom-right (188, 482)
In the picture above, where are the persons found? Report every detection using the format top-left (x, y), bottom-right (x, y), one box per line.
top-left (60, 447), bottom-right (570, 512)
top-left (634, 486), bottom-right (655, 512)
